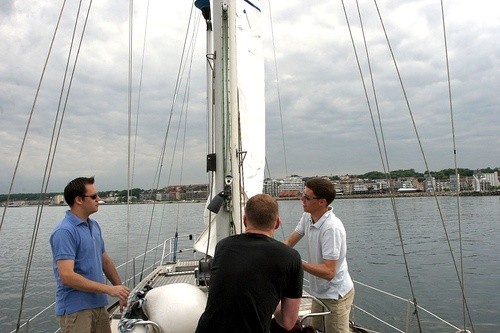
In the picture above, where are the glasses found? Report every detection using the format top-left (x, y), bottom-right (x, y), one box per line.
top-left (302, 192), bottom-right (327, 200)
top-left (77, 192), bottom-right (97, 200)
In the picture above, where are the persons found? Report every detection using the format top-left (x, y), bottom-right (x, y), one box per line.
top-left (283, 177), bottom-right (355, 333)
top-left (49, 177), bottom-right (132, 333)
top-left (197, 194), bottom-right (304, 333)
